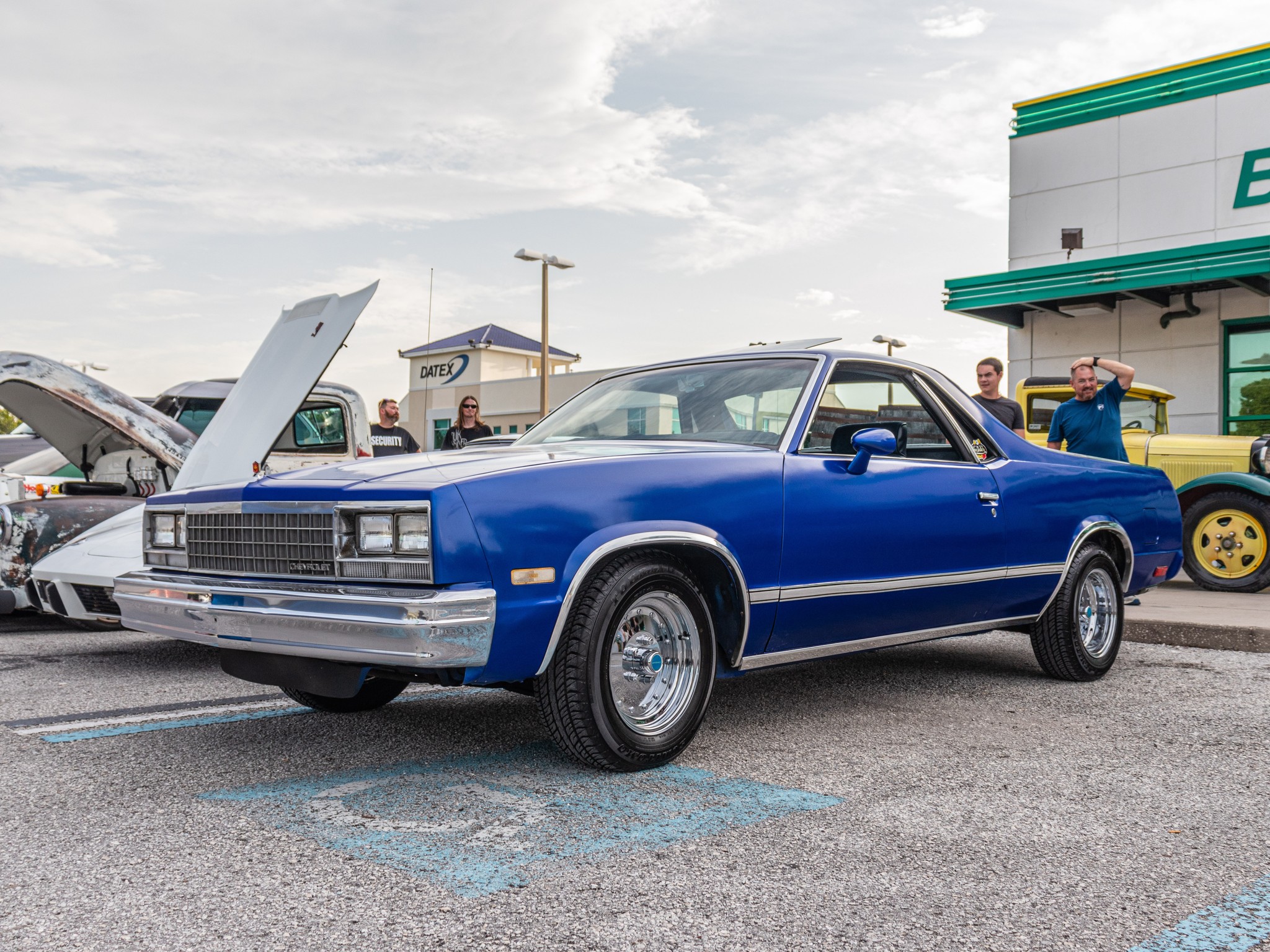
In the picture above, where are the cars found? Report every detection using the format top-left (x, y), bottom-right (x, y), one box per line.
top-left (109, 333), bottom-right (1188, 772)
top-left (0, 278), bottom-right (520, 628)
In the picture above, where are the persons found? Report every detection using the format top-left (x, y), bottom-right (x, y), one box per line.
top-left (370, 398), bottom-right (421, 457)
top-left (439, 395), bottom-right (494, 451)
top-left (1046, 355), bottom-right (1141, 609)
top-left (943, 357), bottom-right (1026, 445)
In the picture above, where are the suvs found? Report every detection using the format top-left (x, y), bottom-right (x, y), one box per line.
top-left (1014, 371), bottom-right (1270, 591)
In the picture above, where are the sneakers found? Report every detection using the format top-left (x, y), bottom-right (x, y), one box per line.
top-left (1124, 595), bottom-right (1141, 605)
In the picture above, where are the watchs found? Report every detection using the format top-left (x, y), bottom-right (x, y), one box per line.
top-left (1093, 356), bottom-right (1101, 367)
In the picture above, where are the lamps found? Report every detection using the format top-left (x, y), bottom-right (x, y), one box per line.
top-left (398, 349), bottom-right (404, 357)
top-left (468, 339), bottom-right (476, 348)
top-left (485, 339), bottom-right (493, 349)
top-left (1060, 228), bottom-right (1084, 260)
top-left (575, 354), bottom-right (579, 362)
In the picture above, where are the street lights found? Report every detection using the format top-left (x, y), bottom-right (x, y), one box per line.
top-left (873, 335), bottom-right (908, 406)
top-left (513, 246), bottom-right (576, 420)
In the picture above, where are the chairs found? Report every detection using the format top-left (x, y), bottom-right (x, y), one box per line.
top-left (831, 421), bottom-right (908, 458)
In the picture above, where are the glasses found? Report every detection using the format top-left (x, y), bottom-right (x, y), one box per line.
top-left (379, 398), bottom-right (394, 409)
top-left (462, 403), bottom-right (477, 409)
